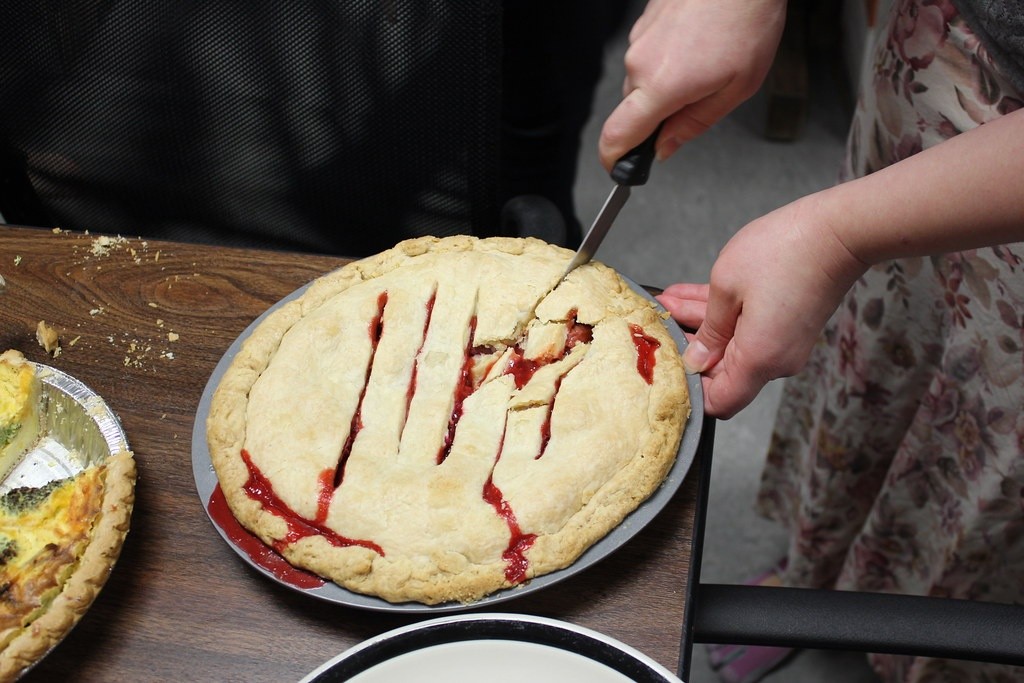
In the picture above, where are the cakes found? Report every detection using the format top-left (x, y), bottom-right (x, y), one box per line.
top-left (0, 350), bottom-right (136, 683)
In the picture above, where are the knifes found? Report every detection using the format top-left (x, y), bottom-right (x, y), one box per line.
top-left (556, 117), bottom-right (669, 288)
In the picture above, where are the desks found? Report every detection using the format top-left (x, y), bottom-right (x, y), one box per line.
top-left (0, 225), bottom-right (716, 683)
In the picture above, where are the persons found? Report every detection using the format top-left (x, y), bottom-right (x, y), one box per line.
top-left (599, 0), bottom-right (1024, 683)
top-left (0, 0), bottom-right (630, 260)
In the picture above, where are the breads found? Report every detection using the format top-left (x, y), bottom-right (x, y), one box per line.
top-left (206, 234), bottom-right (692, 607)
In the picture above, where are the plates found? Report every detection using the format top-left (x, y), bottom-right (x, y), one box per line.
top-left (298, 614), bottom-right (685, 683)
top-left (190, 268), bottom-right (703, 613)
